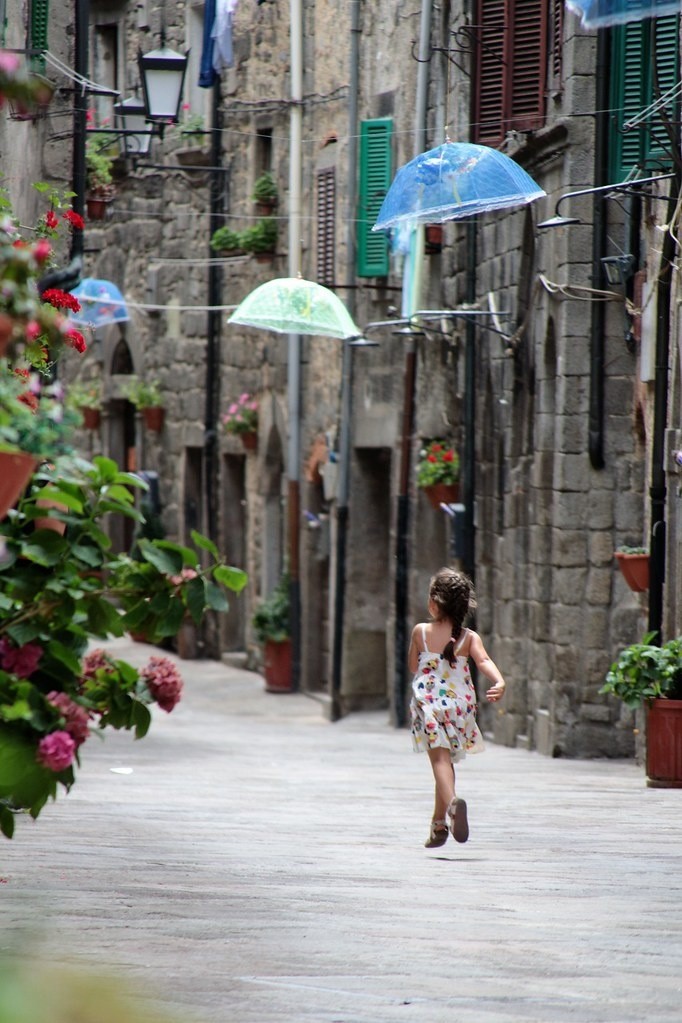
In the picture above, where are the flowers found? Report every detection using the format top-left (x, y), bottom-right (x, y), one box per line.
top-left (168, 103), bottom-right (205, 146)
top-left (219, 393), bottom-right (258, 433)
top-left (415, 441), bottom-right (460, 490)
top-left (0, 178), bottom-right (246, 839)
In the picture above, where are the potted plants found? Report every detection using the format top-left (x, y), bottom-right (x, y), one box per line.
top-left (66, 382), bottom-right (102, 429)
top-left (122, 380), bottom-right (167, 430)
top-left (252, 572), bottom-right (294, 693)
top-left (597, 631), bottom-right (682, 789)
top-left (210, 226), bottom-right (246, 257)
top-left (253, 173), bottom-right (278, 216)
top-left (246, 219), bottom-right (278, 263)
top-left (615, 545), bottom-right (650, 591)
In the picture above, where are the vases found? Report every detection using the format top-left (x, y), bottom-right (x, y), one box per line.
top-left (33, 464), bottom-right (69, 536)
top-left (0, 452), bottom-right (37, 521)
top-left (425, 483), bottom-right (460, 512)
top-left (240, 432), bottom-right (257, 450)
top-left (176, 146), bottom-right (204, 173)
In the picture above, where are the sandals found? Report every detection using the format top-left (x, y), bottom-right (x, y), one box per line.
top-left (424, 819), bottom-right (449, 848)
top-left (448, 797), bottom-right (469, 842)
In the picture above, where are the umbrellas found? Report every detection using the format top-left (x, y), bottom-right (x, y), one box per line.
top-left (63, 276), bottom-right (132, 329)
top-left (225, 273), bottom-right (363, 340)
top-left (371, 127), bottom-right (549, 233)
top-left (564, 0), bottom-right (682, 30)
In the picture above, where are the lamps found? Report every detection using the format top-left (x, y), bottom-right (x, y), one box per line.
top-left (537, 172), bottom-right (677, 228)
top-left (600, 254), bottom-right (634, 286)
top-left (84, 26), bottom-right (191, 141)
top-left (113, 83), bottom-right (229, 172)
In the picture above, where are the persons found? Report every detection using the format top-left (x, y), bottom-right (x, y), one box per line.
top-left (408, 569), bottom-right (506, 849)
